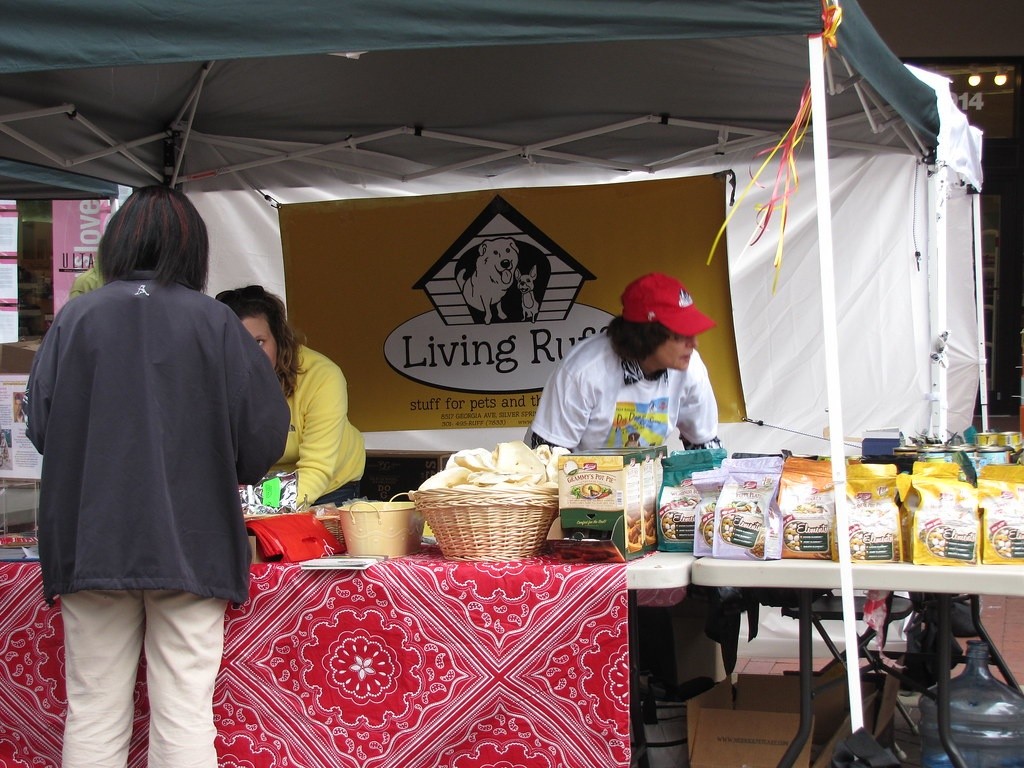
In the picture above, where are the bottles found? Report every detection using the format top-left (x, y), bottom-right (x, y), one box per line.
top-left (919, 640), bottom-right (1024, 768)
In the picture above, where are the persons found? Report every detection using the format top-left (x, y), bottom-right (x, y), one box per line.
top-left (70, 249), bottom-right (105, 300)
top-left (14, 393), bottom-right (25, 423)
top-left (22, 184), bottom-right (291, 768)
top-left (215, 285), bottom-right (366, 509)
top-left (531, 270), bottom-right (723, 454)
top-left (1, 433), bottom-right (9, 461)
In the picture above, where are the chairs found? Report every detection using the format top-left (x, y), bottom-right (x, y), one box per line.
top-left (779, 591), bottom-right (920, 760)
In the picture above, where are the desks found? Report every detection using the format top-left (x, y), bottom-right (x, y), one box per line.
top-left (0, 531), bottom-right (687, 768)
top-left (689, 556), bottom-right (1024, 768)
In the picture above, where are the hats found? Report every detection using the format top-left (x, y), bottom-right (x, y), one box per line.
top-left (621, 272), bottom-right (717, 336)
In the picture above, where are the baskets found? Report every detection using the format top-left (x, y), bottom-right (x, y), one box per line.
top-left (408, 488), bottom-right (558, 561)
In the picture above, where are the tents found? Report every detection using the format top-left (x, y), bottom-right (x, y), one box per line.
top-left (0, 0), bottom-right (941, 768)
top-left (110, 63), bottom-right (989, 643)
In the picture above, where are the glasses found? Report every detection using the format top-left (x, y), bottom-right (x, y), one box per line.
top-left (215, 286), bottom-right (271, 305)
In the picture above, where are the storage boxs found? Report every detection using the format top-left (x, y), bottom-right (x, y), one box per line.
top-left (624, 445), bottom-right (668, 500)
top-left (583, 446), bottom-right (657, 554)
top-left (687, 671), bottom-right (899, 768)
top-left (558, 451), bottom-right (643, 564)
